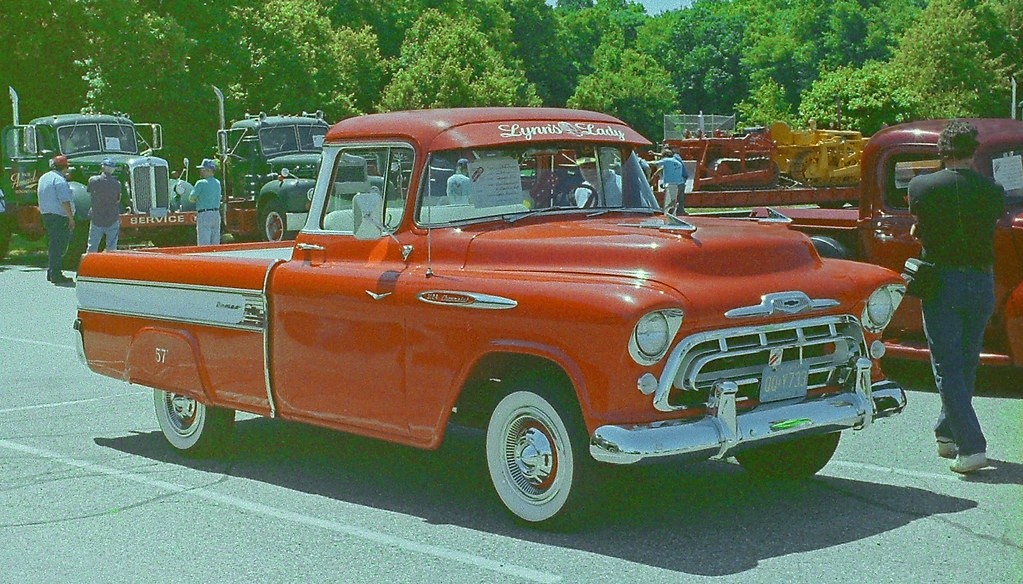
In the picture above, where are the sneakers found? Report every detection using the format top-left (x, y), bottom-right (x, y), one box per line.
top-left (935, 436), bottom-right (956, 457)
top-left (950, 451), bottom-right (987, 473)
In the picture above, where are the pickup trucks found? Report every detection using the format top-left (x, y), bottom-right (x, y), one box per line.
top-left (70, 107), bottom-right (907, 527)
top-left (695, 118), bottom-right (1023, 384)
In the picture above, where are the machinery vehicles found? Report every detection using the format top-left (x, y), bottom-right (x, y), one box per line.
top-left (625, 114), bottom-right (866, 191)
top-left (202, 82), bottom-right (395, 244)
top-left (0, 85), bottom-right (199, 251)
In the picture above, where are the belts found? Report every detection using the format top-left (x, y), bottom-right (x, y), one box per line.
top-left (198, 209), bottom-right (219, 212)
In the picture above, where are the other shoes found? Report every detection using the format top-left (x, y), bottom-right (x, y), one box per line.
top-left (47, 270), bottom-right (51, 280)
top-left (51, 272), bottom-right (73, 283)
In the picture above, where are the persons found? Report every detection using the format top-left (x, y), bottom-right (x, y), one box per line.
top-left (37, 156), bottom-right (73, 283)
top-left (88, 159), bottom-right (121, 252)
top-left (189, 159), bottom-right (221, 247)
top-left (575, 146), bottom-right (622, 208)
top-left (648, 147), bottom-right (688, 213)
top-left (646, 149), bottom-right (682, 214)
top-left (907, 120), bottom-right (1004, 473)
top-left (446, 159), bottom-right (470, 205)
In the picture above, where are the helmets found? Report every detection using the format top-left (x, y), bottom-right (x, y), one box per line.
top-left (53, 156), bottom-right (67, 165)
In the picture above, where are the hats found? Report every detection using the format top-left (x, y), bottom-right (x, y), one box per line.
top-left (458, 158), bottom-right (469, 168)
top-left (575, 146), bottom-right (600, 165)
top-left (101, 160), bottom-right (115, 167)
top-left (196, 158), bottom-right (214, 169)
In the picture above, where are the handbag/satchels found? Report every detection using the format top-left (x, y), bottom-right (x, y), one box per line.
top-left (901, 257), bottom-right (942, 300)
